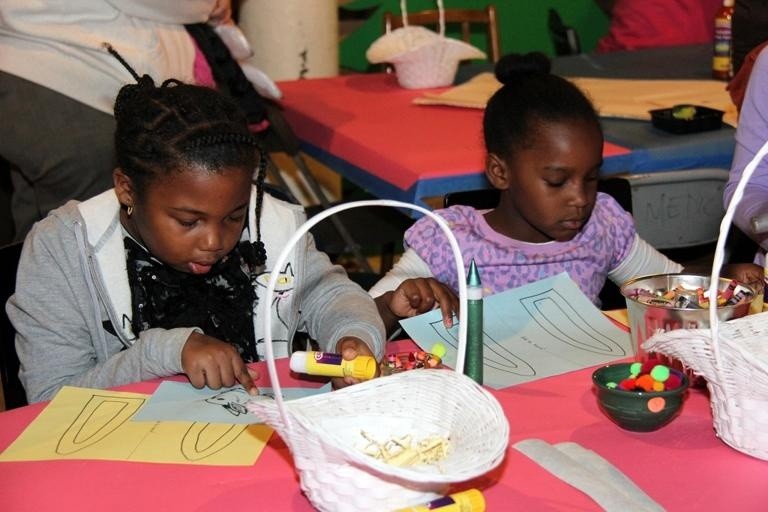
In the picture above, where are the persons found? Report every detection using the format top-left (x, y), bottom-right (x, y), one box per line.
top-left (367, 53), bottom-right (765, 339)
top-left (595, 1), bottom-right (725, 75)
top-left (0, 0), bottom-right (233, 244)
top-left (7, 77), bottom-right (386, 406)
top-left (722, 45), bottom-right (768, 264)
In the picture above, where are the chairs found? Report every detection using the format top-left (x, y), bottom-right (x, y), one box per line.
top-left (443, 175), bottom-right (635, 309)
top-left (383, 5), bottom-right (502, 74)
top-left (624, 168), bottom-right (735, 268)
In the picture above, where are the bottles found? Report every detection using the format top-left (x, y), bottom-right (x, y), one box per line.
top-left (712, 0), bottom-right (736, 79)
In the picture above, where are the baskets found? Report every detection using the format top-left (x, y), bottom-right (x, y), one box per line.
top-left (366, 0), bottom-right (487, 90)
top-left (640, 144), bottom-right (767, 462)
top-left (242, 199), bottom-right (510, 511)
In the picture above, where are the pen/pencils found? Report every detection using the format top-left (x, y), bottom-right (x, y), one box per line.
top-left (387, 301), bottom-right (441, 342)
top-left (627, 279), bottom-right (755, 312)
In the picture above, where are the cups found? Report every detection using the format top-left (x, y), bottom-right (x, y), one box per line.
top-left (619, 271), bottom-right (759, 389)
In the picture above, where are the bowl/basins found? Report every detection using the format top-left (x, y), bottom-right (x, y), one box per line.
top-left (591, 361), bottom-right (691, 433)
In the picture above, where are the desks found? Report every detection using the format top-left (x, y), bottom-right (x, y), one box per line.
top-left (273, 59), bottom-right (738, 228)
top-left (2, 307), bottom-right (767, 512)
